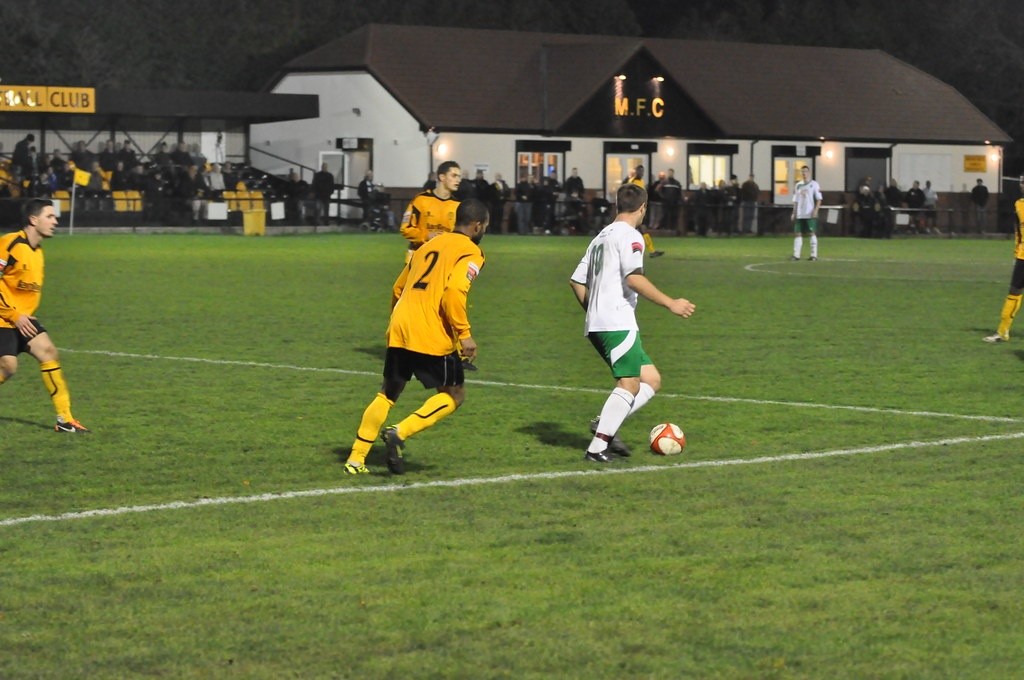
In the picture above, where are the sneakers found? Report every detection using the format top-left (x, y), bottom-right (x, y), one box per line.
top-left (55, 418), bottom-right (90, 435)
top-left (342, 461), bottom-right (370, 475)
top-left (982, 333), bottom-right (1010, 343)
top-left (381, 424), bottom-right (406, 476)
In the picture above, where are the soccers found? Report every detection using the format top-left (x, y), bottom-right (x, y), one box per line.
top-left (648, 423), bottom-right (686, 456)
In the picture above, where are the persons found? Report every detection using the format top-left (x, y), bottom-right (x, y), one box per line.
top-left (787, 165), bottom-right (823, 261)
top-left (400, 160), bottom-right (478, 371)
top-left (971, 178), bottom-right (989, 232)
top-left (1, 130), bottom-right (334, 231)
top-left (569, 185), bottom-right (697, 464)
top-left (357, 169), bottom-right (395, 231)
top-left (0, 198), bottom-right (92, 434)
top-left (421, 168), bottom-right (760, 239)
top-left (855, 175), bottom-right (942, 238)
top-left (982, 175), bottom-right (1024, 343)
top-left (621, 165), bottom-right (665, 258)
top-left (342, 199), bottom-right (489, 477)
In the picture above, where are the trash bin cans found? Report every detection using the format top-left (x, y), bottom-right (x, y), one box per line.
top-left (242, 210), bottom-right (267, 236)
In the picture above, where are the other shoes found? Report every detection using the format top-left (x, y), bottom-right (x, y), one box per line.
top-left (808, 256), bottom-right (817, 261)
top-left (788, 256), bottom-right (799, 260)
top-left (462, 359), bottom-right (479, 371)
top-left (585, 450), bottom-right (614, 462)
top-left (906, 227), bottom-right (941, 235)
top-left (649, 251), bottom-right (664, 259)
top-left (590, 422), bottom-right (632, 458)
